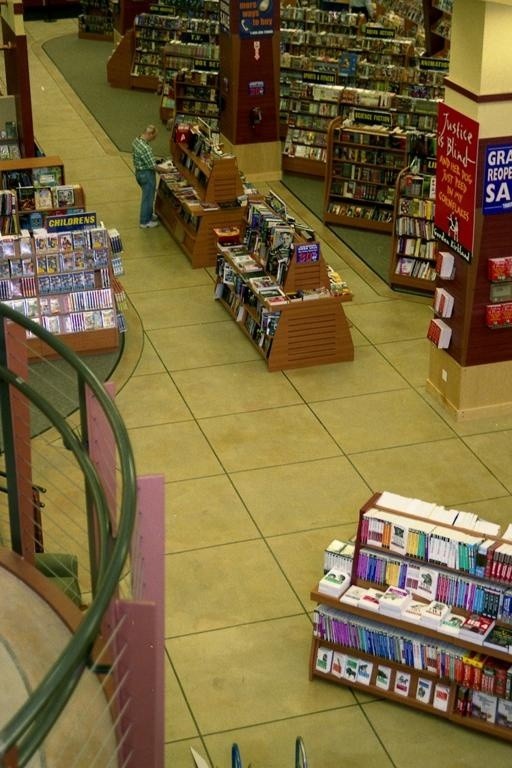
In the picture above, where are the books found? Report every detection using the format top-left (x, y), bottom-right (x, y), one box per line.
top-left (215, 190), bottom-right (344, 351)
top-left (310, 487), bottom-right (510, 740)
top-left (397, 173), bottom-right (438, 281)
top-left (1, 123), bottom-right (125, 361)
top-left (156, 118), bottom-right (258, 228)
top-left (487, 257), bottom-right (512, 327)
top-left (426, 251), bottom-right (455, 348)
top-left (78, 0), bottom-right (117, 33)
top-left (279, 0), bottom-right (453, 223)
top-left (130, 0), bottom-right (222, 131)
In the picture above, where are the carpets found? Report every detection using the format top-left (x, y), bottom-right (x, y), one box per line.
top-left (0, 326), bottom-right (125, 448)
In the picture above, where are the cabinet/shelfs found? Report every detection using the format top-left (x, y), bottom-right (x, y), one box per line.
top-left (154, 119), bottom-right (252, 271)
top-left (321, 115), bottom-right (405, 236)
top-left (38, 1), bottom-right (452, 175)
top-left (0, 220), bottom-right (130, 361)
top-left (0, 156), bottom-right (65, 186)
top-left (1, 95), bottom-right (21, 162)
top-left (305, 493), bottom-right (510, 744)
top-left (388, 165), bottom-right (437, 294)
top-left (213, 188), bottom-right (357, 371)
top-left (0, 182), bottom-right (88, 234)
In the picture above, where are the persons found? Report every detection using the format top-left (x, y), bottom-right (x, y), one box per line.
top-left (132, 125), bottom-right (170, 228)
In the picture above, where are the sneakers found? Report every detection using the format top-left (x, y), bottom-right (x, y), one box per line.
top-left (139, 213), bottom-right (160, 228)
